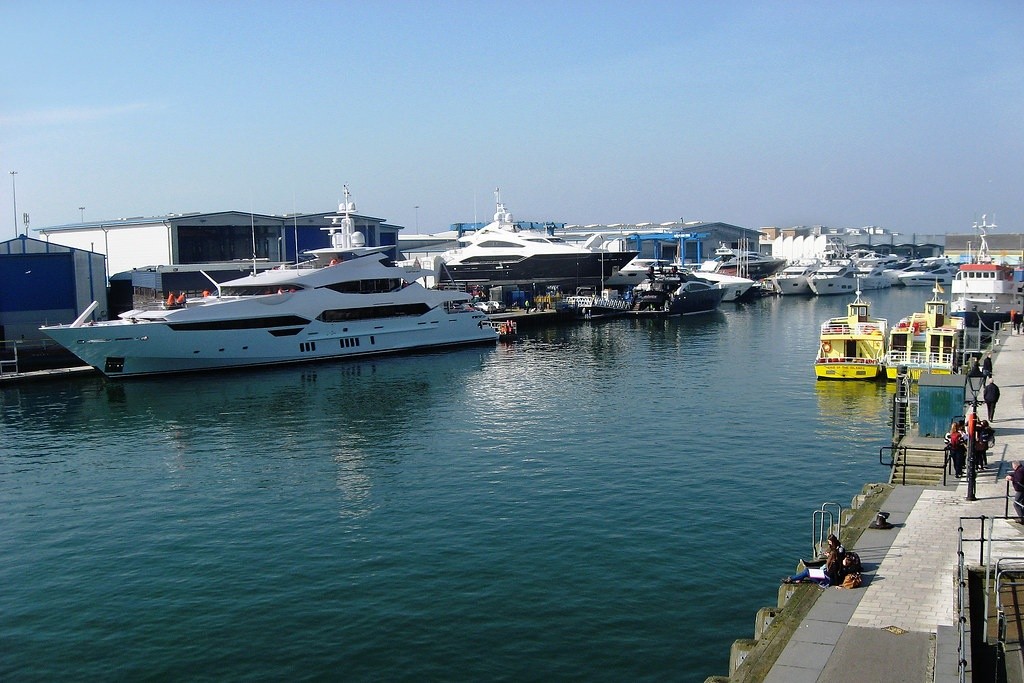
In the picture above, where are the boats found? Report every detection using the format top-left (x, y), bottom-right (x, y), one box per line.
top-left (604, 258), bottom-right (756, 303)
top-left (813, 302), bottom-right (889, 380)
top-left (883, 299), bottom-right (967, 381)
top-left (625, 273), bottom-right (729, 317)
top-left (39, 243), bottom-right (500, 380)
top-left (701, 242), bottom-right (788, 282)
top-left (438, 207), bottom-right (641, 301)
top-left (949, 263), bottom-right (1024, 329)
top-left (773, 252), bottom-right (960, 296)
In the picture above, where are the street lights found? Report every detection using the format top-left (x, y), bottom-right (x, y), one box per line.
top-left (966, 361), bottom-right (987, 501)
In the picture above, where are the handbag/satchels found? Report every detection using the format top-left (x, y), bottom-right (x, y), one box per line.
top-left (840, 573), bottom-right (863, 590)
top-left (988, 436), bottom-right (995, 448)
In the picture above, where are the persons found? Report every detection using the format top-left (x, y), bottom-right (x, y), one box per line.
top-left (776, 532), bottom-right (852, 589)
top-left (262, 256), bottom-right (342, 294)
top-left (1013, 310), bottom-right (1023, 335)
top-left (525, 299), bottom-right (530, 313)
top-left (201, 288), bottom-right (210, 297)
top-left (167, 292), bottom-right (186, 311)
top-left (946, 352), bottom-right (1024, 527)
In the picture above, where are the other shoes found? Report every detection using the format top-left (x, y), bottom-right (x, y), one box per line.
top-left (800, 558), bottom-right (809, 568)
top-left (781, 576), bottom-right (798, 584)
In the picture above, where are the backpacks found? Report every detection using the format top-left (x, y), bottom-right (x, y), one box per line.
top-left (950, 433), bottom-right (960, 450)
top-left (975, 440), bottom-right (985, 451)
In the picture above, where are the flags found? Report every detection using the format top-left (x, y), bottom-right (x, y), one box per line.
top-left (936, 282), bottom-right (945, 294)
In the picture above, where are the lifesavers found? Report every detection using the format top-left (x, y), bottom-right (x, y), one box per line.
top-left (864, 325), bottom-right (874, 335)
top-left (822, 341), bottom-right (831, 352)
top-left (968, 412), bottom-right (974, 440)
